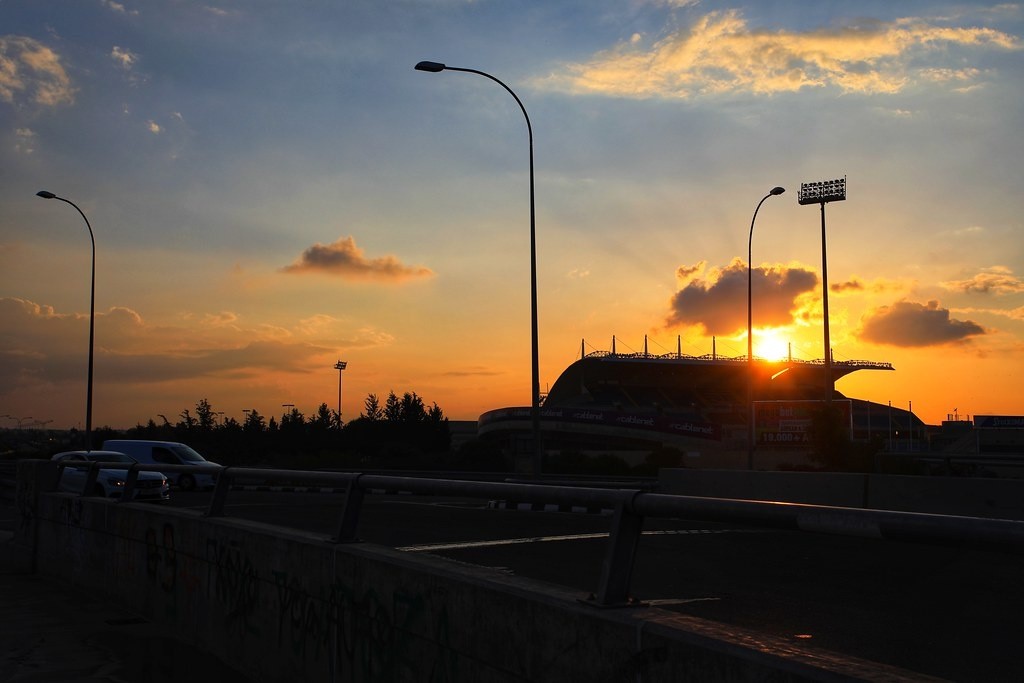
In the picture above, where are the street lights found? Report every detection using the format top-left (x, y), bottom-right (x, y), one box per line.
top-left (334, 359), bottom-right (348, 423)
top-left (217, 412), bottom-right (225, 417)
top-left (746, 186), bottom-right (785, 470)
top-left (283, 404), bottom-right (295, 413)
top-left (242, 409), bottom-right (251, 421)
top-left (413, 60), bottom-right (544, 478)
top-left (36, 190), bottom-right (97, 452)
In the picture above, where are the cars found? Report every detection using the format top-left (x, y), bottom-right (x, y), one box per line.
top-left (48, 451), bottom-right (170, 504)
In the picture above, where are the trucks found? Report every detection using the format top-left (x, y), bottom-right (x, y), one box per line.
top-left (101, 439), bottom-right (224, 494)
